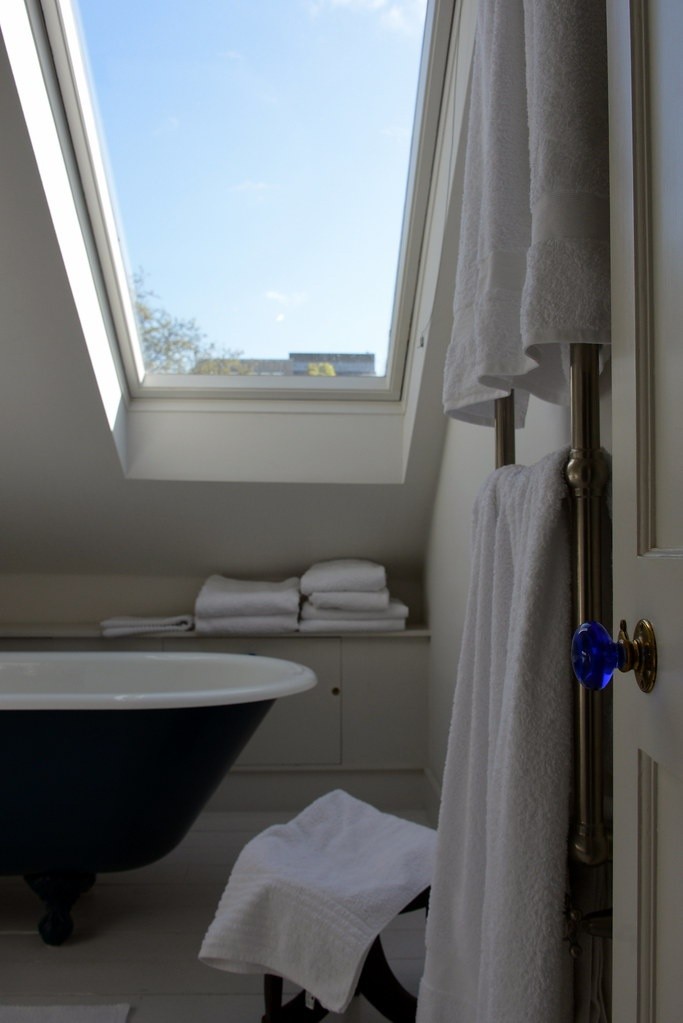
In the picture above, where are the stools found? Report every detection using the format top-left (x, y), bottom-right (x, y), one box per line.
top-left (259, 840), bottom-right (432, 1023)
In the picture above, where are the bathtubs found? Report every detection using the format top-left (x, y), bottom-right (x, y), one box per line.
top-left (0, 651), bottom-right (318, 946)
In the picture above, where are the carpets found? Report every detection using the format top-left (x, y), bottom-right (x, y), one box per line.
top-left (0, 1003), bottom-right (132, 1023)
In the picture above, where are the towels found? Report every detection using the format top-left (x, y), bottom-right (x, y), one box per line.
top-left (100, 614), bottom-right (194, 637)
top-left (196, 790), bottom-right (438, 1014)
top-left (416, 443), bottom-right (571, 1023)
top-left (443, 0), bottom-right (610, 429)
top-left (300, 559), bottom-right (409, 631)
top-left (194, 574), bottom-right (299, 632)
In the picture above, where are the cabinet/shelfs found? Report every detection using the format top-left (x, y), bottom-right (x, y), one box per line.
top-left (163, 638), bottom-right (432, 768)
